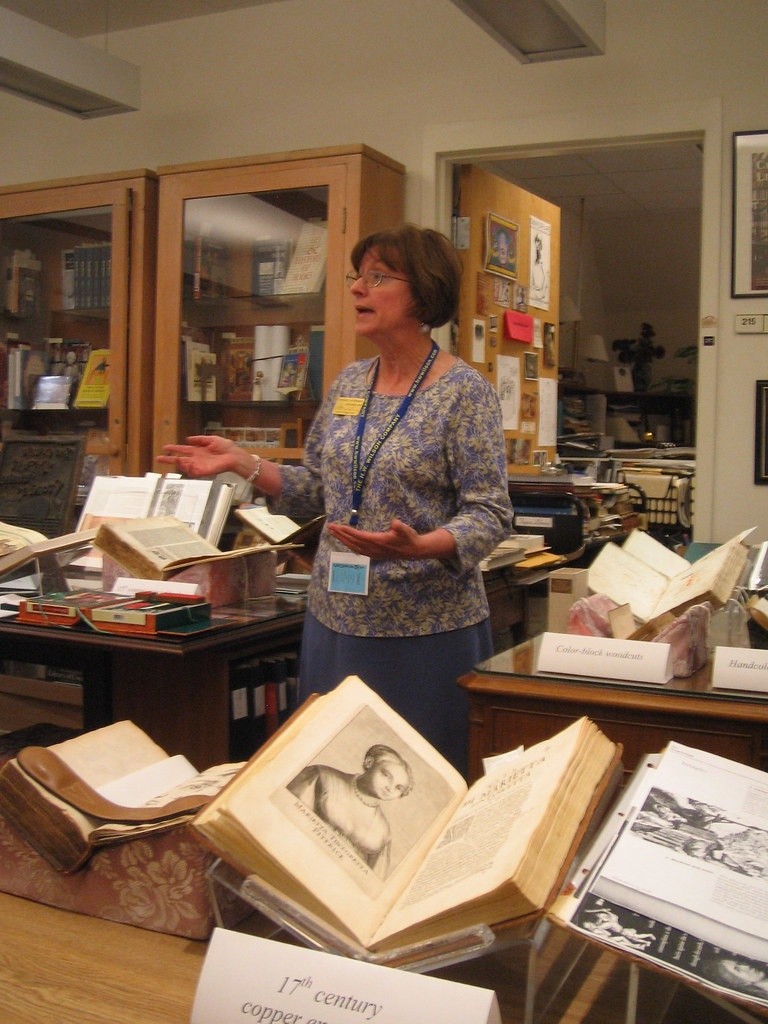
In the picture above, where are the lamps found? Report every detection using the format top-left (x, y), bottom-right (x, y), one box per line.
top-left (559, 297), bottom-right (610, 388)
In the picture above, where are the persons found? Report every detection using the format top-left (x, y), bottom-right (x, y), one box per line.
top-left (158, 220), bottom-right (515, 791)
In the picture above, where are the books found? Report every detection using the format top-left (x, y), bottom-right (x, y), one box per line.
top-left (0, 332), bottom-right (110, 409)
top-left (507, 396), bottom-right (696, 556)
top-left (0, 719), bottom-right (248, 876)
top-left (189, 673), bottom-right (624, 968)
top-left (68, 474), bottom-right (238, 582)
top-left (252, 216), bottom-right (329, 307)
top-left (0, 420), bottom-right (108, 540)
top-left (183, 223), bottom-right (229, 307)
top-left (0, 245), bottom-right (43, 320)
top-left (275, 345), bottom-right (310, 395)
top-left (221, 336), bottom-right (255, 402)
top-left (0, 521), bottom-right (101, 579)
top-left (278, 572), bottom-right (311, 595)
top-left (234, 506), bottom-right (327, 546)
top-left (180, 321), bottom-right (216, 402)
top-left (60, 243), bottom-right (110, 313)
top-left (585, 526), bottom-right (758, 623)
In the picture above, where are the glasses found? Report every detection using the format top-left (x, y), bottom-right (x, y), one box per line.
top-left (346, 270), bottom-right (412, 287)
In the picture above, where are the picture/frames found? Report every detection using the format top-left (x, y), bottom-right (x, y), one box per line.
top-left (730, 130), bottom-right (768, 298)
top-left (753, 380), bottom-right (768, 486)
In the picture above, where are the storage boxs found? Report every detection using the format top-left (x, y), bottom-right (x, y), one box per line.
top-left (547, 567), bottom-right (588, 631)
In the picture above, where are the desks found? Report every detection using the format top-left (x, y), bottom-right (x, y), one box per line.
top-left (0, 892), bottom-right (680, 1024)
top-left (557, 388), bottom-right (696, 449)
top-left (0, 565), bottom-right (525, 777)
top-left (456, 630), bottom-right (768, 789)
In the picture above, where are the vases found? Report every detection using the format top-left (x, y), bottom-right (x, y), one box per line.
top-left (632, 360), bottom-right (651, 393)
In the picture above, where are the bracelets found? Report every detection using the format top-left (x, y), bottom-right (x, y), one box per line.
top-left (246, 454), bottom-right (262, 481)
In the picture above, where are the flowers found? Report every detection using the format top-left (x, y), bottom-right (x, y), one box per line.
top-left (612, 322), bottom-right (665, 366)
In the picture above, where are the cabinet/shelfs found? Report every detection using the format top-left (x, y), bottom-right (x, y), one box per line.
top-left (0, 167), bottom-right (159, 504)
top-left (153, 143), bottom-right (405, 479)
